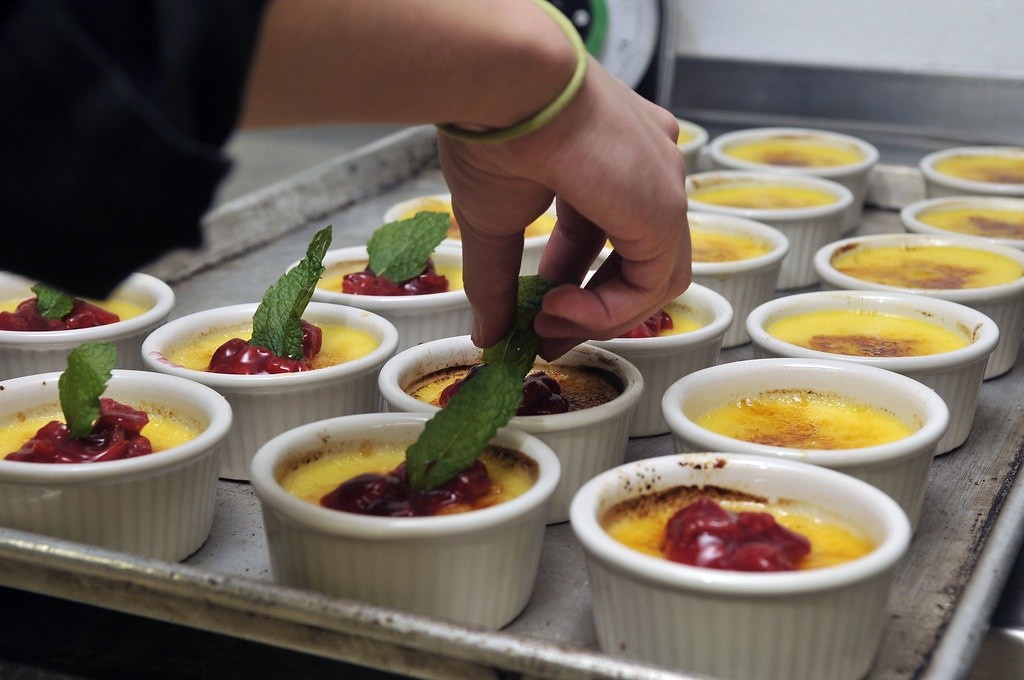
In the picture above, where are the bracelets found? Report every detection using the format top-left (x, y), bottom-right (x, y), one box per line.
top-left (434, 0), bottom-right (588, 145)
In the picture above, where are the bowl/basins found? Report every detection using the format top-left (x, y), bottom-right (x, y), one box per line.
top-left (142, 301), bottom-right (398, 480)
top-left (567, 454), bottom-right (911, 680)
top-left (687, 171), bottom-right (854, 290)
top-left (920, 146), bottom-right (1024, 198)
top-left (287, 244), bottom-right (474, 357)
top-left (676, 118), bottom-right (708, 172)
top-left (0, 271), bottom-right (175, 380)
top-left (386, 194), bottom-right (555, 277)
top-left (660, 359), bottom-right (949, 539)
top-left (584, 281), bottom-right (732, 435)
top-left (250, 412), bottom-right (560, 632)
top-left (115, 303), bottom-right (116, 306)
top-left (814, 235), bottom-right (1024, 381)
top-left (746, 290), bottom-right (1000, 456)
top-left (0, 369), bottom-right (234, 563)
top-left (901, 197), bottom-right (1024, 249)
top-left (383, 339), bottom-right (645, 528)
top-left (709, 127), bottom-right (879, 233)
top-left (595, 212), bottom-right (788, 348)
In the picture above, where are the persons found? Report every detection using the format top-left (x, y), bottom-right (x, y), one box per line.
top-left (0, 0), bottom-right (694, 363)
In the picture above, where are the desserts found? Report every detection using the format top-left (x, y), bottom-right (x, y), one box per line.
top-left (0, 113), bottom-right (1024, 680)
